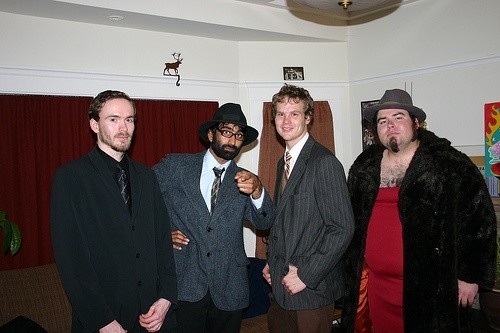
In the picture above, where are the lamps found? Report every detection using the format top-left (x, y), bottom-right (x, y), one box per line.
top-left (287, 0), bottom-right (401, 26)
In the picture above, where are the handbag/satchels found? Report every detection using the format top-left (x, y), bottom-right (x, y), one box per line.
top-left (243, 258), bottom-right (271, 319)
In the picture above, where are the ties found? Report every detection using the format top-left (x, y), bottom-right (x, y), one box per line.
top-left (280, 151), bottom-right (291, 198)
top-left (210, 167), bottom-right (225, 215)
top-left (117, 162), bottom-right (132, 217)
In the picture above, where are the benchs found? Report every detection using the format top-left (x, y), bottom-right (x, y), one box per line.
top-left (0, 263), bottom-right (270, 333)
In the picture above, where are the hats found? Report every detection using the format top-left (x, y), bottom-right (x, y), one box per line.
top-left (199, 102), bottom-right (259, 148)
top-left (365, 89), bottom-right (427, 125)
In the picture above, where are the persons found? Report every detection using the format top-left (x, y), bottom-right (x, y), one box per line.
top-left (341, 89), bottom-right (497, 333)
top-left (51, 90), bottom-right (178, 333)
top-left (152, 102), bottom-right (277, 333)
top-left (262, 82), bottom-right (356, 333)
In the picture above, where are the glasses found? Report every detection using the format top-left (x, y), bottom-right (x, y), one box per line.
top-left (216, 128), bottom-right (245, 141)
